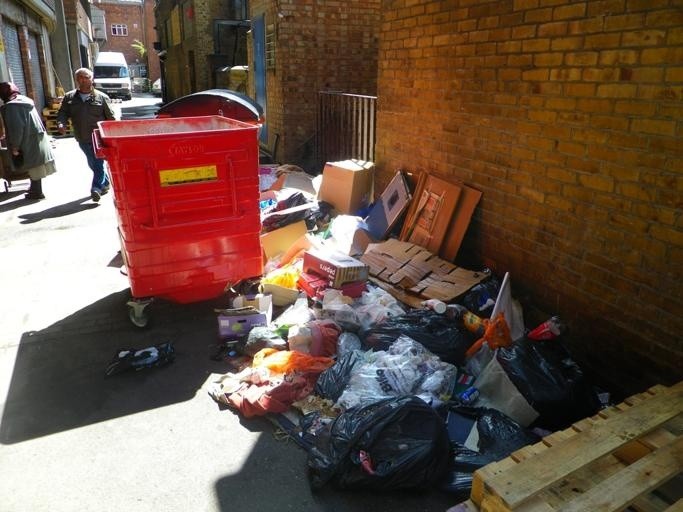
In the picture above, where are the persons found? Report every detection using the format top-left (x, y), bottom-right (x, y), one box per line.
top-left (55, 68), bottom-right (115, 201)
top-left (0, 82), bottom-right (56, 201)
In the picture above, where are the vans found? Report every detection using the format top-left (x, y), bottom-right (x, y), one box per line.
top-left (93, 52), bottom-right (132, 100)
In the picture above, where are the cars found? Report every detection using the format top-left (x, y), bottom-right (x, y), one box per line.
top-left (152, 78), bottom-right (161, 96)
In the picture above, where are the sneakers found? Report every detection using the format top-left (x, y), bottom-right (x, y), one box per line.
top-left (100, 185), bottom-right (109, 195)
top-left (91, 190), bottom-right (100, 202)
top-left (25, 193), bottom-right (45, 199)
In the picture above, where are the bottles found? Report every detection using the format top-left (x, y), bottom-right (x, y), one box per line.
top-left (528, 316), bottom-right (565, 339)
top-left (457, 386), bottom-right (480, 405)
top-left (445, 304), bottom-right (485, 335)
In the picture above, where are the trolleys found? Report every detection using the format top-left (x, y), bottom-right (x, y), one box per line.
top-left (0, 136), bottom-right (30, 193)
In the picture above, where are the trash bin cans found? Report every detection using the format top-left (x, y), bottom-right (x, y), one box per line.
top-left (154, 89), bottom-right (266, 141)
top-left (90, 115), bottom-right (266, 331)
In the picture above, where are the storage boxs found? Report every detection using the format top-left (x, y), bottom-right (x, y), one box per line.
top-left (317, 158), bottom-right (375, 215)
